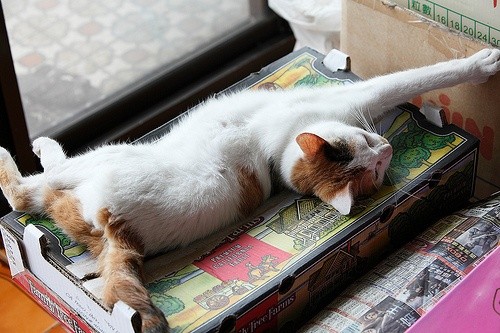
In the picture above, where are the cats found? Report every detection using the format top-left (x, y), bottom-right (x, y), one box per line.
top-left (0, 48), bottom-right (500, 333)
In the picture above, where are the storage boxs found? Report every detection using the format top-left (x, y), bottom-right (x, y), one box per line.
top-left (0, 46), bottom-right (480, 333)
top-left (340, 0), bottom-right (500, 201)
top-left (393, 0), bottom-right (500, 48)
